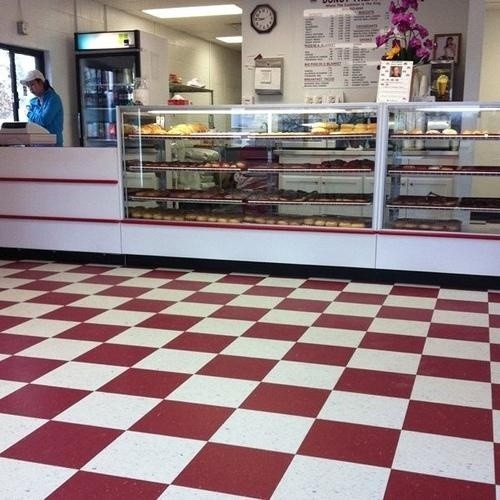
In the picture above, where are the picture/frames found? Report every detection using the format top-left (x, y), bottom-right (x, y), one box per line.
top-left (434, 33), bottom-right (461, 64)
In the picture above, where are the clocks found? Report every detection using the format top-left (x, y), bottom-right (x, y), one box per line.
top-left (250, 4), bottom-right (277, 33)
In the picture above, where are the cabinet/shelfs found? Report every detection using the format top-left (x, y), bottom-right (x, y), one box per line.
top-left (117, 103), bottom-right (500, 280)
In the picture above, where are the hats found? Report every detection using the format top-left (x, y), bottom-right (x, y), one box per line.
top-left (20, 70), bottom-right (45, 85)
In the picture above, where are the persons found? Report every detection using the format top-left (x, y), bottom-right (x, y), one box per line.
top-left (18, 68), bottom-right (64, 149)
top-left (391, 66), bottom-right (400, 77)
top-left (443, 37), bottom-right (456, 59)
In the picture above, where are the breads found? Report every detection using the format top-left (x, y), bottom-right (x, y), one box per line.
top-left (124, 121), bottom-right (489, 231)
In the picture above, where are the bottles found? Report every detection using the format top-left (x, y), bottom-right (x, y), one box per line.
top-left (98, 119), bottom-right (115, 135)
top-left (133, 77), bottom-right (149, 104)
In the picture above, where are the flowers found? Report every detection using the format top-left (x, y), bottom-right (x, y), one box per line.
top-left (372, 0), bottom-right (438, 69)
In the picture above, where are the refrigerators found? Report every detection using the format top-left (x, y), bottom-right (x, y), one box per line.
top-left (72, 29), bottom-right (172, 145)
